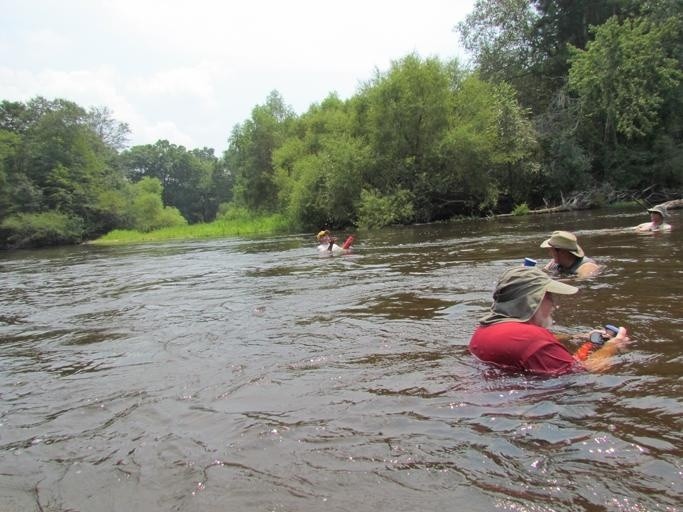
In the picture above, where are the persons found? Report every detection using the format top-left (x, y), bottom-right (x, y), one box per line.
top-left (539, 231), bottom-right (601, 283)
top-left (632, 203), bottom-right (672, 236)
top-left (465, 265), bottom-right (632, 418)
top-left (316, 229), bottom-right (351, 258)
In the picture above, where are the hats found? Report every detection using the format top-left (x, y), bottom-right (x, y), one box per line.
top-left (646, 204), bottom-right (672, 218)
top-left (316, 230), bottom-right (330, 240)
top-left (540, 231), bottom-right (585, 258)
top-left (479, 266), bottom-right (579, 324)
